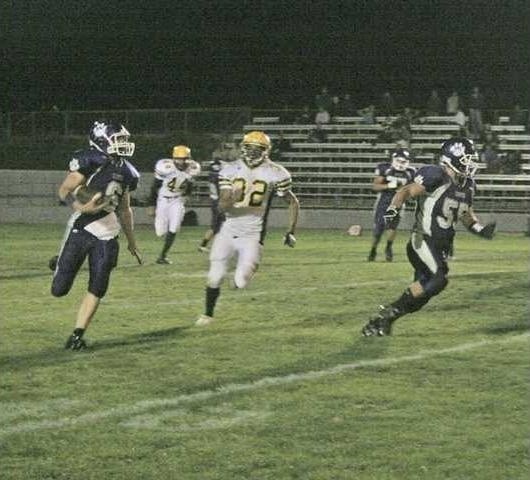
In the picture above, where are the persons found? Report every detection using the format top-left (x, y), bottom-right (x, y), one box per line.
top-left (48, 118), bottom-right (142, 351)
top-left (149, 144), bottom-right (201, 265)
top-left (366, 148), bottom-right (417, 261)
top-left (193, 130), bottom-right (300, 326)
top-left (361, 135), bottom-right (496, 336)
top-left (268, 84), bottom-right (530, 174)
top-left (198, 156), bottom-right (226, 253)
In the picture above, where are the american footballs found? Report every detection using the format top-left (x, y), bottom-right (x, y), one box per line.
top-left (72, 185), bottom-right (103, 204)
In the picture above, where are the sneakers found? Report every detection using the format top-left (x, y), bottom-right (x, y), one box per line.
top-left (362, 317), bottom-right (393, 337)
top-left (194, 314), bottom-right (214, 326)
top-left (65, 334), bottom-right (92, 353)
top-left (155, 257), bottom-right (174, 265)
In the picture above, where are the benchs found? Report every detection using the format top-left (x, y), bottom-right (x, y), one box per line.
top-left (178, 113), bottom-right (530, 212)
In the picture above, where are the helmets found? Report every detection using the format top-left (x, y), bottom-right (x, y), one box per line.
top-left (438, 135), bottom-right (478, 178)
top-left (89, 119), bottom-right (137, 158)
top-left (172, 145), bottom-right (191, 159)
top-left (239, 130), bottom-right (272, 168)
top-left (391, 148), bottom-right (410, 173)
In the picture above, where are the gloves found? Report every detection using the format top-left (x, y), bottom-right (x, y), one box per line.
top-left (469, 220), bottom-right (496, 239)
top-left (387, 179), bottom-right (403, 190)
top-left (282, 232), bottom-right (297, 249)
top-left (381, 206), bottom-right (399, 230)
